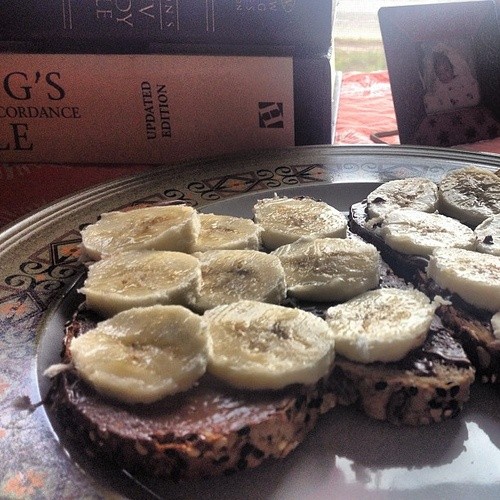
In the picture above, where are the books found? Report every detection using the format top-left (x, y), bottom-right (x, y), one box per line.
top-left (1, 0), bottom-right (342, 167)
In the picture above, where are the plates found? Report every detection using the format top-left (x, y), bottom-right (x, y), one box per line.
top-left (35, 183), bottom-right (499, 500)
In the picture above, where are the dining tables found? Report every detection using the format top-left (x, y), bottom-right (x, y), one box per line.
top-left (0, 69), bottom-right (500, 232)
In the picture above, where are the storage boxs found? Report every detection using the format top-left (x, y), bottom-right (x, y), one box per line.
top-left (0, 0), bottom-right (343, 164)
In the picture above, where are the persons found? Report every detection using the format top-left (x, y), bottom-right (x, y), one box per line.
top-left (423, 47), bottom-right (479, 114)
